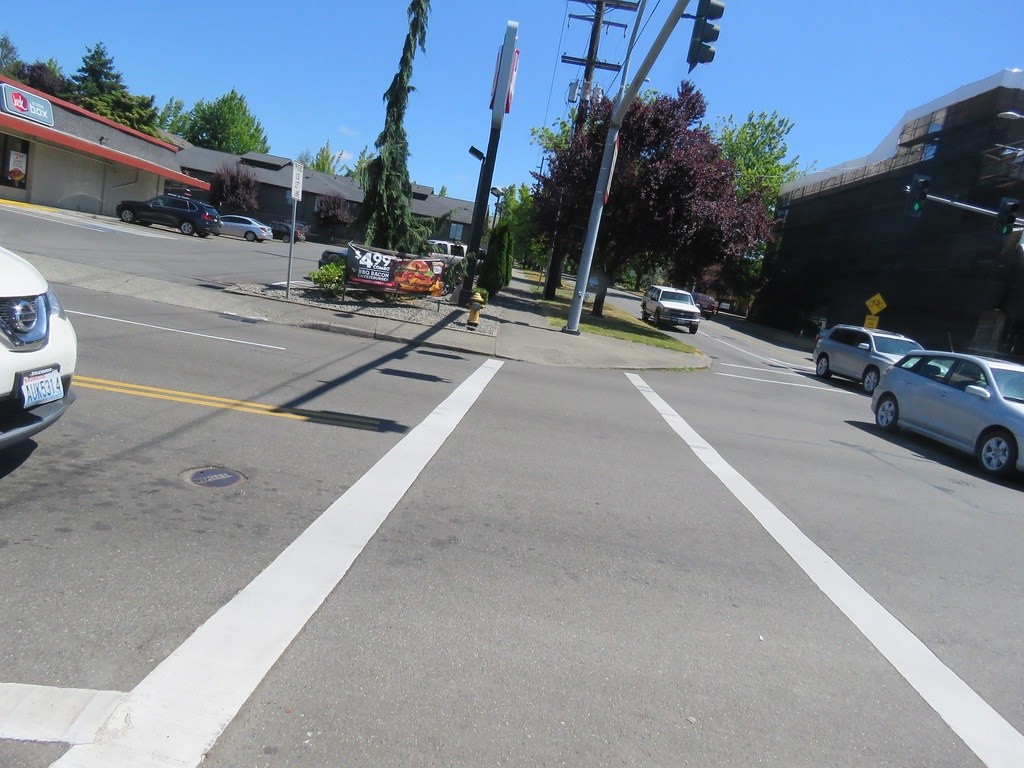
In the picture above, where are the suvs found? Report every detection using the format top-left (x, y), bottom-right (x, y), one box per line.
top-left (692, 293), bottom-right (717, 319)
top-left (114, 194), bottom-right (222, 239)
top-left (640, 285), bottom-right (701, 334)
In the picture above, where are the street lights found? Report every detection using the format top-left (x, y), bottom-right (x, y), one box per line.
top-left (686, 0), bottom-right (724, 73)
top-left (465, 147), bottom-right (486, 257)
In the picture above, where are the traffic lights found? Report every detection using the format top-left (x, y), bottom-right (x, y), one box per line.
top-left (903, 174), bottom-right (932, 219)
top-left (994, 197), bottom-right (1018, 236)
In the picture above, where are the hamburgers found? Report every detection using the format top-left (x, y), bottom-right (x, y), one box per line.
top-left (393, 261), bottom-right (435, 295)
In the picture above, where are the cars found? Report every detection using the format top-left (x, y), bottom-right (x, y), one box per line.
top-left (213, 215), bottom-right (273, 243)
top-left (870, 349), bottom-right (1024, 477)
top-left (812, 324), bottom-right (924, 397)
top-left (264, 222), bottom-right (302, 244)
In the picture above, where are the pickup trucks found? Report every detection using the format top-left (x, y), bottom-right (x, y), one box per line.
top-left (426, 239), bottom-right (485, 276)
